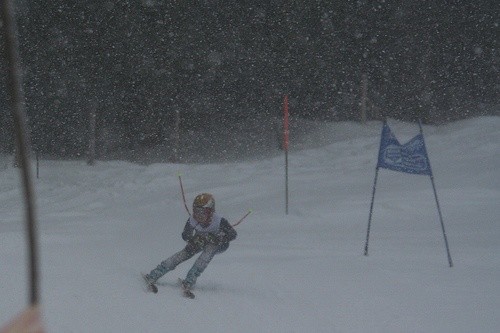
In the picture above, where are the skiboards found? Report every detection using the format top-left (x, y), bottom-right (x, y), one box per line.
top-left (140, 269), bottom-right (159, 293)
top-left (177, 275), bottom-right (195, 300)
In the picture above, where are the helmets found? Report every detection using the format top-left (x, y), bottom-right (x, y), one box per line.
top-left (193, 192), bottom-right (214, 210)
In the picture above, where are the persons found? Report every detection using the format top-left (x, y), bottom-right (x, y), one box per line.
top-left (141, 193), bottom-right (238, 299)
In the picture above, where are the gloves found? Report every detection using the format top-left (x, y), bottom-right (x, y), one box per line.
top-left (191, 236), bottom-right (203, 249)
top-left (206, 232), bottom-right (219, 246)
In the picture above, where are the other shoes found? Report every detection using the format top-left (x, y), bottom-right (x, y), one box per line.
top-left (143, 274), bottom-right (158, 294)
top-left (182, 280), bottom-right (195, 299)
